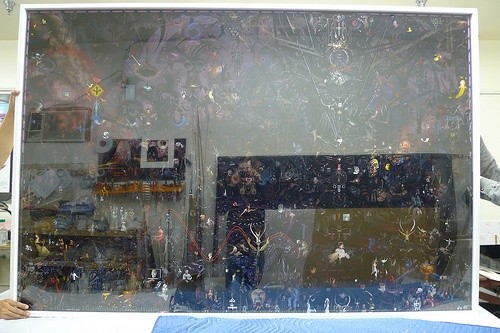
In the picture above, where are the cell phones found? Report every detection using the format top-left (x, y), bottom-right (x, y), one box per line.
top-left (239, 170), bottom-right (251, 178)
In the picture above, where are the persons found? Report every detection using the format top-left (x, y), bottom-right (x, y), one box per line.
top-left (0, 289), bottom-right (31, 319)
top-left (216, 156), bottom-right (272, 295)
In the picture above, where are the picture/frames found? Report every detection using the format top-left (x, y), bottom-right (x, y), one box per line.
top-left (7, 3), bottom-right (481, 321)
